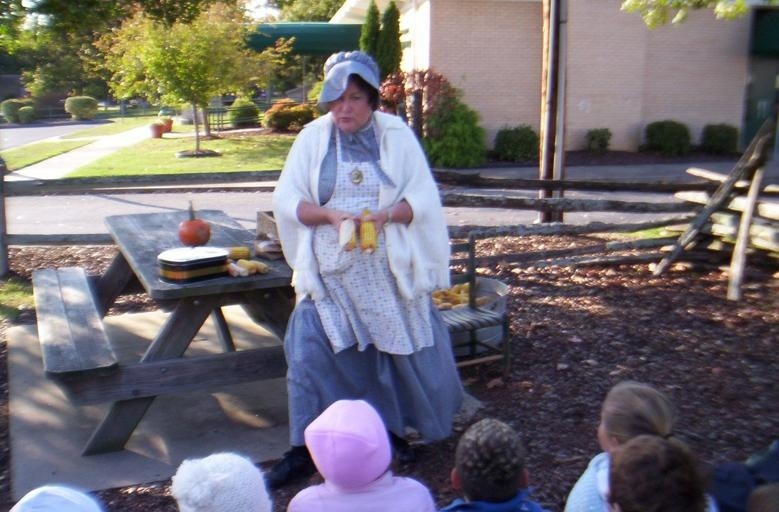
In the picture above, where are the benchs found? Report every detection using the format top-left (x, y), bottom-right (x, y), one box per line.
top-left (31, 264), bottom-right (117, 407)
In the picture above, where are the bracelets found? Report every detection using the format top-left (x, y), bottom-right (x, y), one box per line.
top-left (384, 206), bottom-right (392, 226)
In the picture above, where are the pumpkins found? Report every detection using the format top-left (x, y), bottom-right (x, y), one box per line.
top-left (178, 199), bottom-right (210, 245)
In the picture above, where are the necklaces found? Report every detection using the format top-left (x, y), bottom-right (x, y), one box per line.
top-left (342, 134), bottom-right (372, 184)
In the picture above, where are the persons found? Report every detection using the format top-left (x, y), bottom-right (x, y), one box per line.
top-left (602, 432), bottom-right (710, 511)
top-left (703, 439), bottom-right (779, 510)
top-left (269, 49), bottom-right (464, 480)
top-left (439, 416), bottom-right (546, 511)
top-left (560, 377), bottom-right (719, 511)
top-left (284, 397), bottom-right (438, 512)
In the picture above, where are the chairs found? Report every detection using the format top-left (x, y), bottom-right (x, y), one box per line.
top-left (428, 230), bottom-right (510, 387)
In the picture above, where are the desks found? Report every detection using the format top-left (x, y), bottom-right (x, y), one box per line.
top-left (79, 208), bottom-right (295, 458)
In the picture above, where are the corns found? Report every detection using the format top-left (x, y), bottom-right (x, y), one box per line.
top-left (340, 207), bottom-right (376, 255)
top-left (432, 281), bottom-right (487, 310)
top-left (229, 246), bottom-right (268, 277)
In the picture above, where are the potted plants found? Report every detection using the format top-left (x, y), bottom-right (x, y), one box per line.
top-left (151, 123), bottom-right (164, 138)
top-left (161, 116), bottom-right (174, 133)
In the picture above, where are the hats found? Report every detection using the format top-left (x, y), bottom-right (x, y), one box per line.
top-left (310, 50), bottom-right (380, 109)
top-left (299, 397), bottom-right (391, 488)
